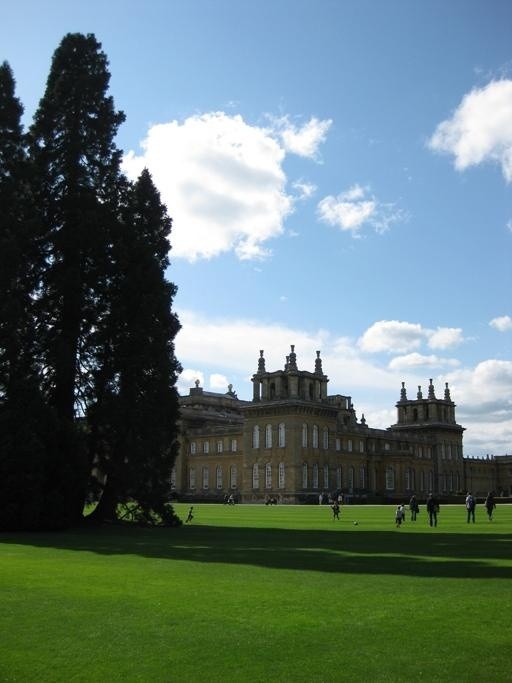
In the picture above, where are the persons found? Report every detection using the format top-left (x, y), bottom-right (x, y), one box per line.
top-left (427, 493), bottom-right (439, 527)
top-left (185, 507), bottom-right (194, 523)
top-left (395, 504), bottom-right (405, 524)
top-left (485, 491), bottom-right (496, 521)
top-left (410, 495), bottom-right (419, 521)
top-left (466, 492), bottom-right (475, 523)
top-left (331, 501), bottom-right (340, 520)
top-left (319, 492), bottom-right (344, 506)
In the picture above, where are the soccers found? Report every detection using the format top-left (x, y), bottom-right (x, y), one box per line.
top-left (354, 522), bottom-right (357, 525)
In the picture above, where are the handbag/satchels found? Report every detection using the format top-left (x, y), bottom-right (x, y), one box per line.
top-left (415, 508), bottom-right (419, 513)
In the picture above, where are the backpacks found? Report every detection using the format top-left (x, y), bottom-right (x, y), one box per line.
top-left (469, 499), bottom-right (475, 508)
